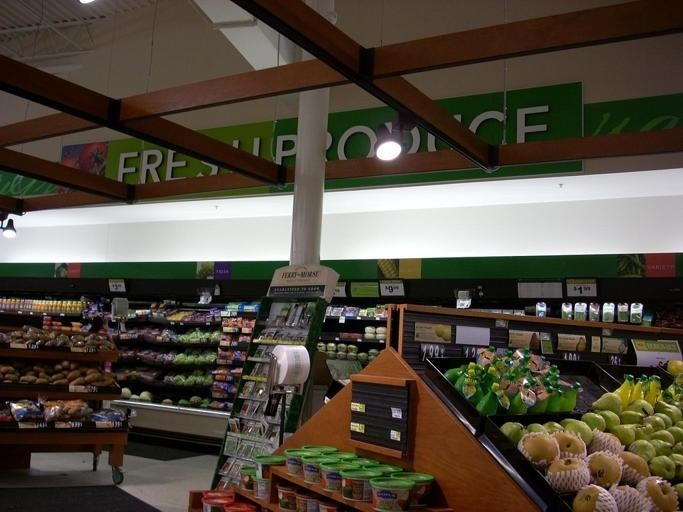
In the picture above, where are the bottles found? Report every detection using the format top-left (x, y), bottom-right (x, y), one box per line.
top-left (613, 374), bottom-right (662, 409)
top-left (448, 345), bottom-right (579, 414)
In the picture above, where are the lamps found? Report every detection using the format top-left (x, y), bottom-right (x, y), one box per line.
top-left (373, 112), bottom-right (417, 161)
top-left (0, 209), bottom-right (26, 238)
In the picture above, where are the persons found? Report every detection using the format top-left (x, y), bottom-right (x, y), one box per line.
top-left (88, 315), bottom-right (112, 410)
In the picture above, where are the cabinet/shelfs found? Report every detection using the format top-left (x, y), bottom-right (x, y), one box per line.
top-left (0, 278), bottom-right (683, 512)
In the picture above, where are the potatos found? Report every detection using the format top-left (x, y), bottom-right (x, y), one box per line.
top-left (1, 361), bottom-right (114, 387)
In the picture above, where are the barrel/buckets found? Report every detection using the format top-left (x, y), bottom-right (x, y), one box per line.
top-left (239, 445), bottom-right (437, 511)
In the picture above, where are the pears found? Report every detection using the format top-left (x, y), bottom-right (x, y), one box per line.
top-left (500, 393), bottom-right (683, 512)
